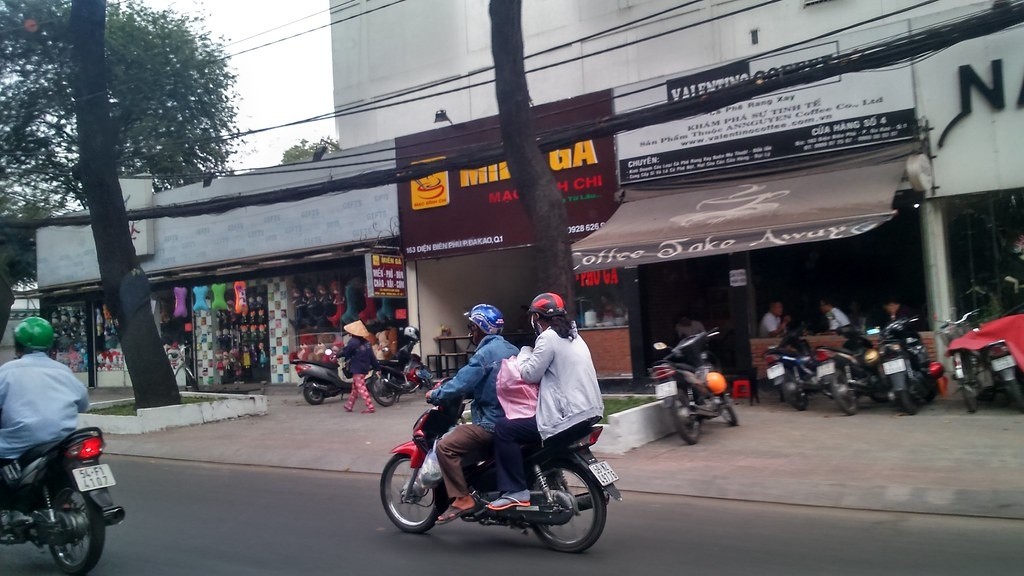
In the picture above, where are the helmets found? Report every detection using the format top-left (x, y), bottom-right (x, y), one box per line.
top-left (705, 372), bottom-right (728, 394)
top-left (14, 317), bottom-right (54, 350)
top-left (404, 326), bottom-right (421, 341)
top-left (464, 304), bottom-right (504, 336)
top-left (529, 293), bottom-right (565, 316)
top-left (927, 362), bottom-right (944, 378)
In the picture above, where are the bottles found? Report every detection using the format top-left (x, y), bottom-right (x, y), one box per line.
top-left (441, 326), bottom-right (451, 337)
top-left (801, 319), bottom-right (808, 336)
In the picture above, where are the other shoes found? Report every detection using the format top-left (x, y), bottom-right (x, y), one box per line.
top-left (344, 404), bottom-right (352, 411)
top-left (361, 409), bottom-right (375, 413)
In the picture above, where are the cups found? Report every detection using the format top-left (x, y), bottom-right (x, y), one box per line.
top-left (616, 318), bottom-right (624, 325)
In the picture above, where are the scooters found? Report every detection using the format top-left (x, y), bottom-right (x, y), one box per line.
top-left (289, 358), bottom-right (353, 405)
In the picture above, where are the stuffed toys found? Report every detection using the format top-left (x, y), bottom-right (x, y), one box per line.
top-left (290, 326), bottom-right (400, 366)
top-left (51, 304), bottom-right (88, 372)
top-left (215, 294), bottom-right (266, 380)
top-left (163, 341), bottom-right (188, 373)
top-left (96, 305), bottom-right (128, 371)
top-left (289, 278), bottom-right (398, 326)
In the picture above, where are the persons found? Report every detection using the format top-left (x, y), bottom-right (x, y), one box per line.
top-left (268, 308), bottom-right (289, 383)
top-left (818, 299), bottom-right (853, 337)
top-left (595, 291), bottom-right (628, 324)
top-left (871, 294), bottom-right (933, 380)
top-left (999, 227), bottom-right (1024, 389)
top-left (194, 311), bottom-right (214, 375)
top-left (425, 302), bottom-right (538, 526)
top-left (757, 300), bottom-right (790, 336)
top-left (0, 316), bottom-right (89, 524)
top-left (335, 319), bottom-right (382, 413)
top-left (487, 293), bottom-right (606, 509)
top-left (674, 312), bottom-right (710, 350)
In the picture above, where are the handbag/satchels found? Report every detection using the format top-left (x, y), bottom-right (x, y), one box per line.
top-left (496, 355), bottom-right (540, 420)
top-left (418, 439), bottom-right (442, 489)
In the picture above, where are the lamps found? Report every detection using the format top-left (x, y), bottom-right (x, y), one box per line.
top-left (202, 171), bottom-right (215, 188)
top-left (312, 138), bottom-right (342, 160)
top-left (434, 109), bottom-right (453, 125)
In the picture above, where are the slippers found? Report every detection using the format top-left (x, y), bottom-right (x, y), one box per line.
top-left (487, 495), bottom-right (530, 510)
top-left (435, 504), bottom-right (475, 525)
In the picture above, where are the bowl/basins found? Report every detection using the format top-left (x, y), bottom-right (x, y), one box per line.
top-left (584, 311), bottom-right (615, 328)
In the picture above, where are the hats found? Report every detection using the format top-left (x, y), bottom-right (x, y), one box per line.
top-left (344, 320), bottom-right (369, 336)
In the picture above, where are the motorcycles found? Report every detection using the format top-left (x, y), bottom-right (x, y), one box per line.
top-left (758, 303), bottom-right (1024, 417)
top-left (645, 325), bottom-right (739, 445)
top-left (0, 426), bottom-right (126, 576)
top-left (377, 376), bottom-right (622, 553)
top-left (367, 324), bottom-right (436, 408)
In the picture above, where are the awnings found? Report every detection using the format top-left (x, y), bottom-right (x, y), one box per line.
top-left (570, 136), bottom-right (906, 276)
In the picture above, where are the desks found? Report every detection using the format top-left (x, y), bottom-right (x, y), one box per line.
top-left (502, 332), bottom-right (535, 347)
top-left (436, 336), bottom-right (472, 378)
top-left (749, 331), bottom-right (938, 406)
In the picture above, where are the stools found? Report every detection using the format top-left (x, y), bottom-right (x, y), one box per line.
top-left (732, 379), bottom-right (751, 400)
top-left (426, 354), bottom-right (448, 378)
top-left (445, 352), bottom-right (469, 377)
